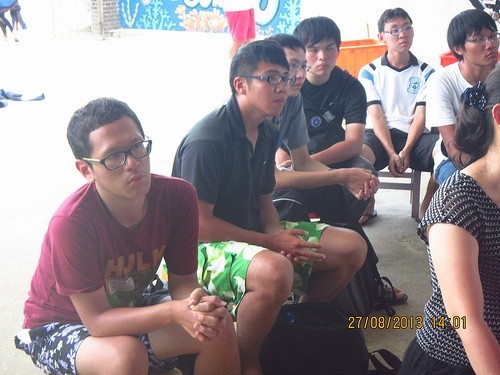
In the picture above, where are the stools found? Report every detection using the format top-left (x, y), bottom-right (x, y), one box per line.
top-left (378, 165), bottom-right (419, 220)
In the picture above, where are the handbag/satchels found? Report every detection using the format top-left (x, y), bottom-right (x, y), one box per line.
top-left (321, 219), bottom-right (380, 316)
top-left (259, 302), bottom-right (369, 375)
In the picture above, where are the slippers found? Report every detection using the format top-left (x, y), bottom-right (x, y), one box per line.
top-left (359, 211), bottom-right (378, 226)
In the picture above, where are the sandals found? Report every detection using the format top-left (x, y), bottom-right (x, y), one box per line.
top-left (382, 283), bottom-right (408, 304)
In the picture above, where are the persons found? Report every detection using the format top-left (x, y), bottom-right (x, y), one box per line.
top-left (14, 98), bottom-right (240, 375)
top-left (359, 7), bottom-right (441, 226)
top-left (276, 16), bottom-right (377, 213)
top-left (171, 38), bottom-right (367, 374)
top-left (265, 31), bottom-right (407, 311)
top-left (403, 59), bottom-right (500, 375)
top-left (417, 9), bottom-right (500, 221)
top-left (223, 0), bottom-right (255, 58)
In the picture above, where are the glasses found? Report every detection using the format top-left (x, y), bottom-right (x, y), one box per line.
top-left (83, 140), bottom-right (152, 170)
top-left (240, 74), bottom-right (296, 87)
top-left (385, 26), bottom-right (413, 36)
top-left (289, 64), bottom-right (311, 72)
top-left (465, 33), bottom-right (500, 45)
